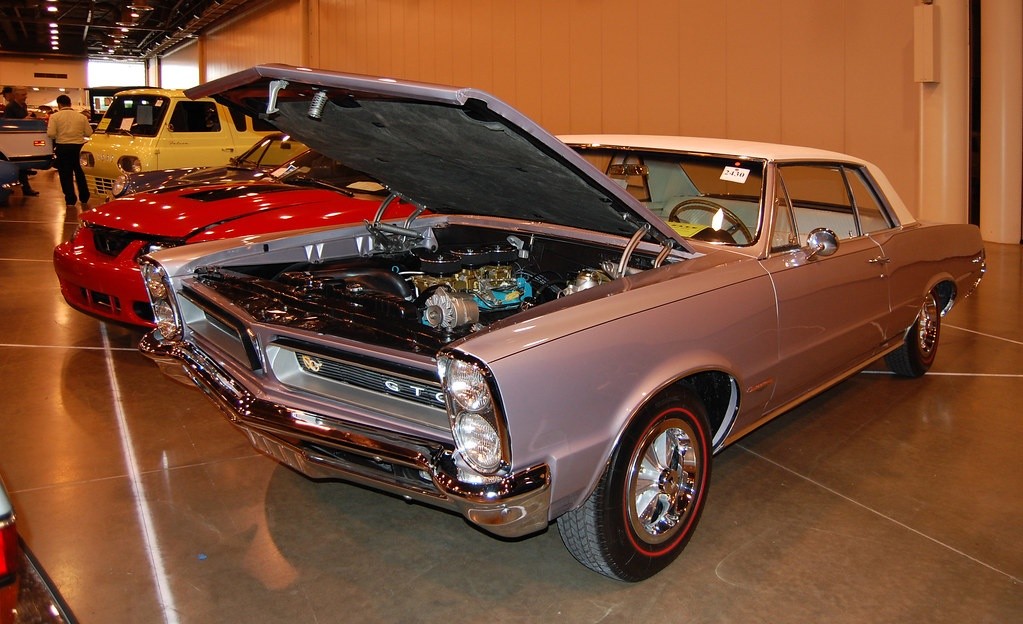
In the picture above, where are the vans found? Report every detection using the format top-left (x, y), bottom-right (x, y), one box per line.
top-left (80, 88), bottom-right (309, 197)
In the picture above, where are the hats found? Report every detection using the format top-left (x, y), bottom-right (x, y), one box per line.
top-left (0, 87), bottom-right (12, 95)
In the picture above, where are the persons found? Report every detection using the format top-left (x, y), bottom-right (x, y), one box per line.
top-left (47, 94), bottom-right (93, 207)
top-left (0, 86), bottom-right (40, 197)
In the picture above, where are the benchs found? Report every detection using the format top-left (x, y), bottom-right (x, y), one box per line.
top-left (663, 196), bottom-right (881, 235)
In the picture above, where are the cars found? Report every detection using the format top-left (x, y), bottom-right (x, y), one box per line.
top-left (138, 63), bottom-right (989, 586)
top-left (51, 134), bottom-right (433, 337)
top-left (0, 118), bottom-right (54, 170)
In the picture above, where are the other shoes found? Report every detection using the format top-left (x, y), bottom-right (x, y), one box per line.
top-left (67, 201), bottom-right (87, 206)
top-left (24, 190), bottom-right (39, 196)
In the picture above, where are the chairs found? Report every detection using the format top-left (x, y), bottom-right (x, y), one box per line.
top-left (657, 216), bottom-right (689, 224)
top-left (729, 226), bottom-right (806, 248)
